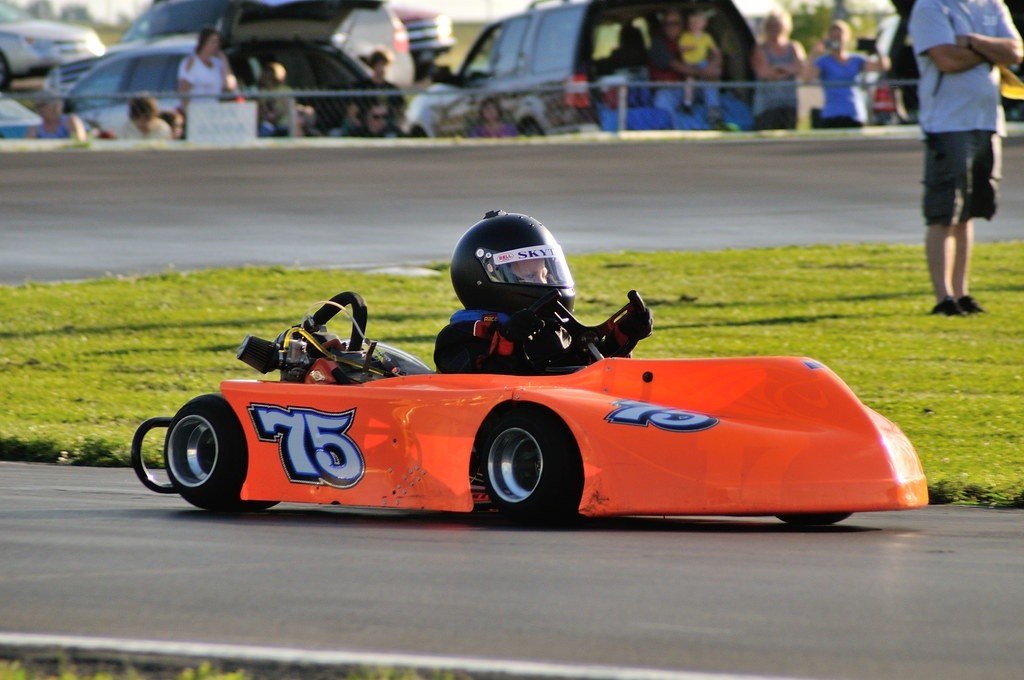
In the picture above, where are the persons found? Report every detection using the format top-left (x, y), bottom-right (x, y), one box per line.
top-left (24, 8), bottom-right (921, 139)
top-left (433, 210), bottom-right (652, 375)
top-left (910, 0), bottom-right (1024, 314)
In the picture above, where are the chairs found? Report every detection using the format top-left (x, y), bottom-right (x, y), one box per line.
top-left (611, 22), bottom-right (645, 68)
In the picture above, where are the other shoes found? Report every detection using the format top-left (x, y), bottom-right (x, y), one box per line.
top-left (930, 296), bottom-right (983, 317)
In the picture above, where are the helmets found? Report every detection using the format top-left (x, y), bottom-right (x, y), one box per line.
top-left (451, 210), bottom-right (575, 316)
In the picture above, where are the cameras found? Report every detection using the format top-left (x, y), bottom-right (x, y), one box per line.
top-left (825, 41), bottom-right (839, 50)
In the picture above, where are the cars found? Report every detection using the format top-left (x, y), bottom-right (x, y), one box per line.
top-left (0, 7), bottom-right (108, 89)
top-left (56, 2), bottom-right (454, 138)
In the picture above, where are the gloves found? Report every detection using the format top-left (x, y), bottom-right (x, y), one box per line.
top-left (499, 307), bottom-right (545, 347)
top-left (618, 309), bottom-right (655, 341)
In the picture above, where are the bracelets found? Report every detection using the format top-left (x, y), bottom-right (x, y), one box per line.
top-left (966, 32), bottom-right (974, 50)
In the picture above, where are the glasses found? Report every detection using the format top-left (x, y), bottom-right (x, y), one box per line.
top-left (372, 114), bottom-right (388, 120)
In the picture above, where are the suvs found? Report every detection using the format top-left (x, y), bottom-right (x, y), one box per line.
top-left (403, 0), bottom-right (759, 134)
top-left (853, 0), bottom-right (1024, 122)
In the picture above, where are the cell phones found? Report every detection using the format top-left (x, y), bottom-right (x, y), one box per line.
top-left (857, 38), bottom-right (875, 50)
